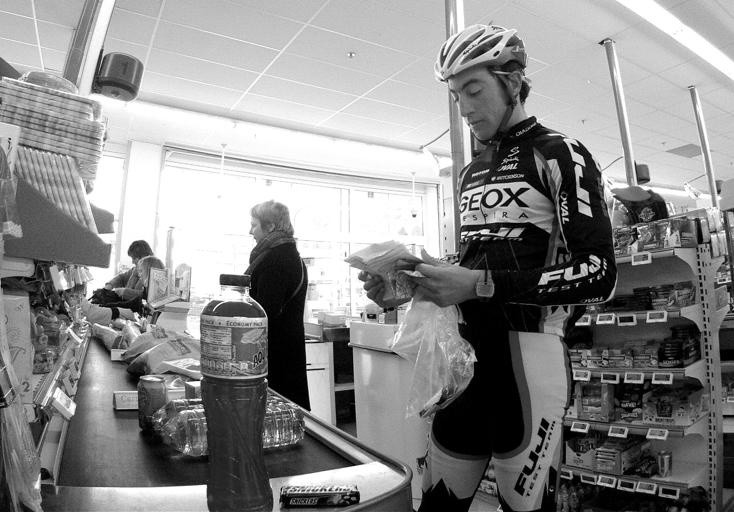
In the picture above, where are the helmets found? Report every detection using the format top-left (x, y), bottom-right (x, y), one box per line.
top-left (433, 23), bottom-right (528, 84)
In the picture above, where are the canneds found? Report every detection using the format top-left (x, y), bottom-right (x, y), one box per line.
top-left (137, 375), bottom-right (167, 430)
top-left (657, 450), bottom-right (672, 477)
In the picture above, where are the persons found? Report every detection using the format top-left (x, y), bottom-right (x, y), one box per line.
top-left (356, 23), bottom-right (619, 512)
top-left (233, 200), bottom-right (311, 413)
top-left (103, 240), bottom-right (155, 300)
top-left (100, 256), bottom-right (168, 325)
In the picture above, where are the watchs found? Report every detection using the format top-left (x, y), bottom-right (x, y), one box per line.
top-left (474, 269), bottom-right (495, 303)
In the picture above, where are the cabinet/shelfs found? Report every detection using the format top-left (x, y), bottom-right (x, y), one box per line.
top-left (561, 244), bottom-right (733, 507)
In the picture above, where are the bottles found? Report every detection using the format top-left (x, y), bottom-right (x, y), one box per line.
top-left (143, 395), bottom-right (306, 458)
top-left (200, 274), bottom-right (274, 512)
